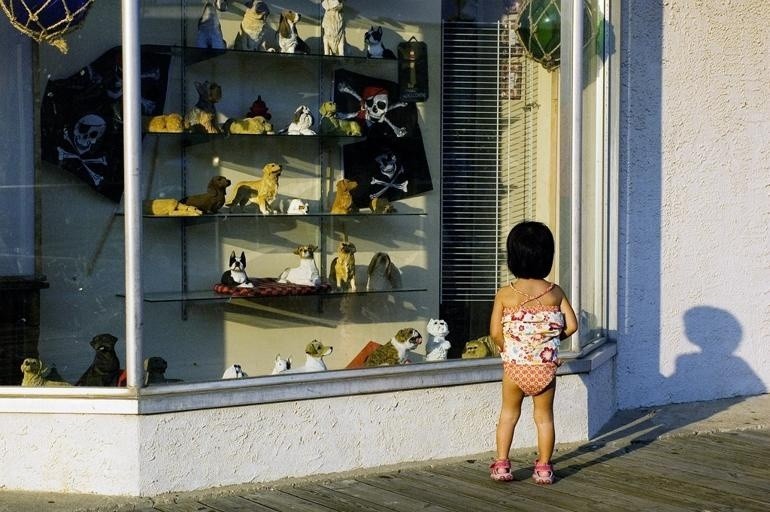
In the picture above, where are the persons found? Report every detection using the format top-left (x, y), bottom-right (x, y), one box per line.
top-left (489, 221), bottom-right (578, 485)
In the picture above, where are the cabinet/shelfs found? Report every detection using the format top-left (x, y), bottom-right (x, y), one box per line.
top-left (114, 0), bottom-right (429, 323)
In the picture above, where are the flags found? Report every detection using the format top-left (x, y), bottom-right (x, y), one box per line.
top-left (331, 68), bottom-right (434, 208)
top-left (40, 43), bottom-right (172, 206)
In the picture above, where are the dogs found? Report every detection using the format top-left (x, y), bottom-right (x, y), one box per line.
top-left (365, 25), bottom-right (395, 59)
top-left (330, 239), bottom-right (358, 293)
top-left (460, 336), bottom-right (500, 359)
top-left (20, 357), bottom-right (72, 387)
top-left (317, 101), bottom-right (362, 137)
top-left (367, 250), bottom-right (402, 291)
top-left (276, 243), bottom-right (322, 289)
top-left (287, 199), bottom-right (309, 216)
top-left (329, 178), bottom-right (358, 214)
top-left (372, 197), bottom-right (397, 214)
top-left (226, 162), bottom-right (283, 215)
top-left (233, 0), bottom-right (276, 53)
top-left (424, 317), bottom-right (452, 362)
top-left (143, 198), bottom-right (203, 217)
top-left (271, 352), bottom-right (294, 377)
top-left (287, 105), bottom-right (316, 136)
top-left (321, 0), bottom-right (345, 56)
top-left (278, 8), bottom-right (311, 54)
top-left (222, 116), bottom-right (273, 135)
top-left (147, 356), bottom-right (181, 385)
top-left (221, 250), bottom-right (254, 290)
top-left (74, 334), bottom-right (121, 386)
top-left (364, 327), bottom-right (423, 367)
top-left (149, 114), bottom-right (181, 131)
top-left (196, 0), bottom-right (227, 50)
top-left (183, 79), bottom-right (221, 134)
top-left (178, 175), bottom-right (232, 213)
top-left (221, 362), bottom-right (248, 379)
top-left (280, 338), bottom-right (333, 374)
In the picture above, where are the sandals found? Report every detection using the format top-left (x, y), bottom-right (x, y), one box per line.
top-left (532, 458), bottom-right (556, 484)
top-left (489, 458), bottom-right (513, 481)
